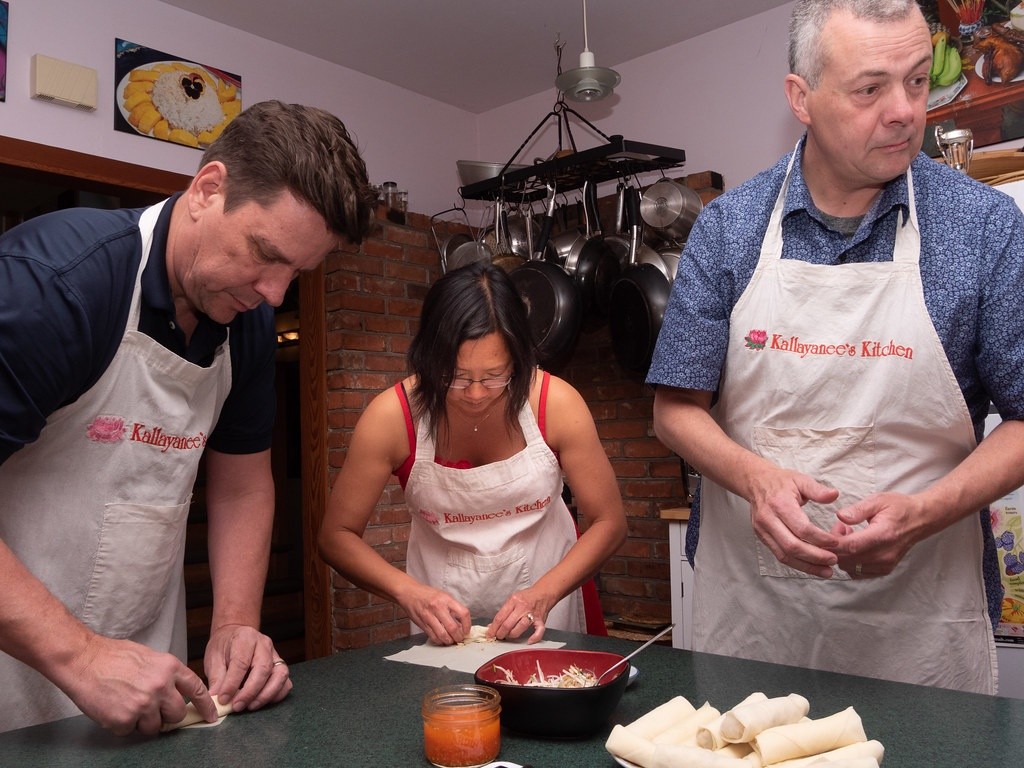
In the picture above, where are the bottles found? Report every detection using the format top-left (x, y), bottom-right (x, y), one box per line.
top-left (959, 20), bottom-right (982, 44)
top-left (382, 182), bottom-right (409, 212)
top-left (423, 684), bottom-right (501, 768)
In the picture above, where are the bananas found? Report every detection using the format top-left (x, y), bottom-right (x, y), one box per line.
top-left (929, 35), bottom-right (962, 89)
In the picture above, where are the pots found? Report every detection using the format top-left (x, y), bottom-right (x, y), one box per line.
top-left (431, 159), bottom-right (702, 368)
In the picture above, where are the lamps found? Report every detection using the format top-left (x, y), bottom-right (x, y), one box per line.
top-left (555, 0), bottom-right (621, 103)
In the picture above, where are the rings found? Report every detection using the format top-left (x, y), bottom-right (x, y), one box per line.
top-left (856, 562), bottom-right (862, 575)
top-left (526, 613), bottom-right (535, 623)
top-left (273, 661), bottom-right (288, 666)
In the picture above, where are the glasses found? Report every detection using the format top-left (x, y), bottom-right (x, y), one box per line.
top-left (442, 365), bottom-right (515, 389)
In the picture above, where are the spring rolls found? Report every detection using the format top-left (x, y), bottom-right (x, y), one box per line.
top-left (158, 689), bottom-right (241, 731)
top-left (605, 691), bottom-right (886, 768)
top-left (384, 625), bottom-right (568, 675)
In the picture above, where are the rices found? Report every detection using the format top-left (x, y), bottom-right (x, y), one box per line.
top-left (153, 69), bottom-right (226, 132)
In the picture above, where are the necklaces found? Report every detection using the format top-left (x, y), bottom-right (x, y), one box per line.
top-left (446, 393), bottom-right (505, 432)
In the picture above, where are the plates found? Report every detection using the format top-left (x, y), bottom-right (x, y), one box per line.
top-left (926, 73), bottom-right (968, 113)
top-left (613, 755), bottom-right (641, 768)
top-left (975, 55), bottom-right (1024, 83)
top-left (116, 59), bottom-right (242, 150)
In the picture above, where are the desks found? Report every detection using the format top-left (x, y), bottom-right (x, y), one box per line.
top-left (0, 617), bottom-right (1024, 768)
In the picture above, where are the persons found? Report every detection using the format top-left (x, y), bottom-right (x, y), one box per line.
top-left (645, 0), bottom-right (1024, 696)
top-left (0, 101), bottom-right (380, 736)
top-left (317, 261), bottom-right (628, 645)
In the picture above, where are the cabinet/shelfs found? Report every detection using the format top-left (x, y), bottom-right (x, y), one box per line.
top-left (659, 505), bottom-right (695, 649)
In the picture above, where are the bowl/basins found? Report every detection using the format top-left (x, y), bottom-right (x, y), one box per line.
top-left (474, 649), bottom-right (629, 740)
